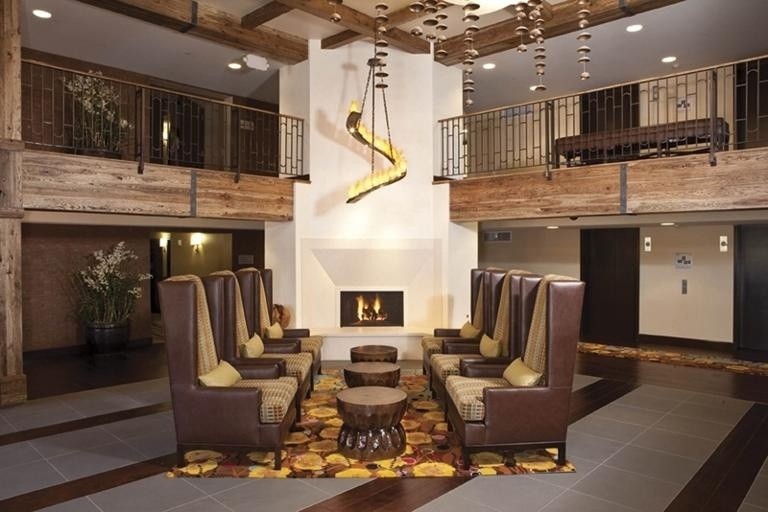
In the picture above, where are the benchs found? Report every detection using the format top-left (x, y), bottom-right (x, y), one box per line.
top-left (555, 118), bottom-right (729, 168)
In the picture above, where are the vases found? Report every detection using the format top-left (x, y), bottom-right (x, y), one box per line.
top-left (77, 148), bottom-right (122, 160)
top-left (86, 322), bottom-right (130, 356)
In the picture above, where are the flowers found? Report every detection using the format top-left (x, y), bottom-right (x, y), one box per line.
top-left (65, 239), bottom-right (154, 328)
top-left (62, 72), bottom-right (137, 153)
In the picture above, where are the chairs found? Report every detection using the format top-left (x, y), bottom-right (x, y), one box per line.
top-left (421, 266), bottom-right (586, 471)
top-left (158, 266), bottom-right (323, 470)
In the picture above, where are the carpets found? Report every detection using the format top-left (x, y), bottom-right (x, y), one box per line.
top-left (577, 342), bottom-right (767, 381)
top-left (166, 370), bottom-right (577, 477)
